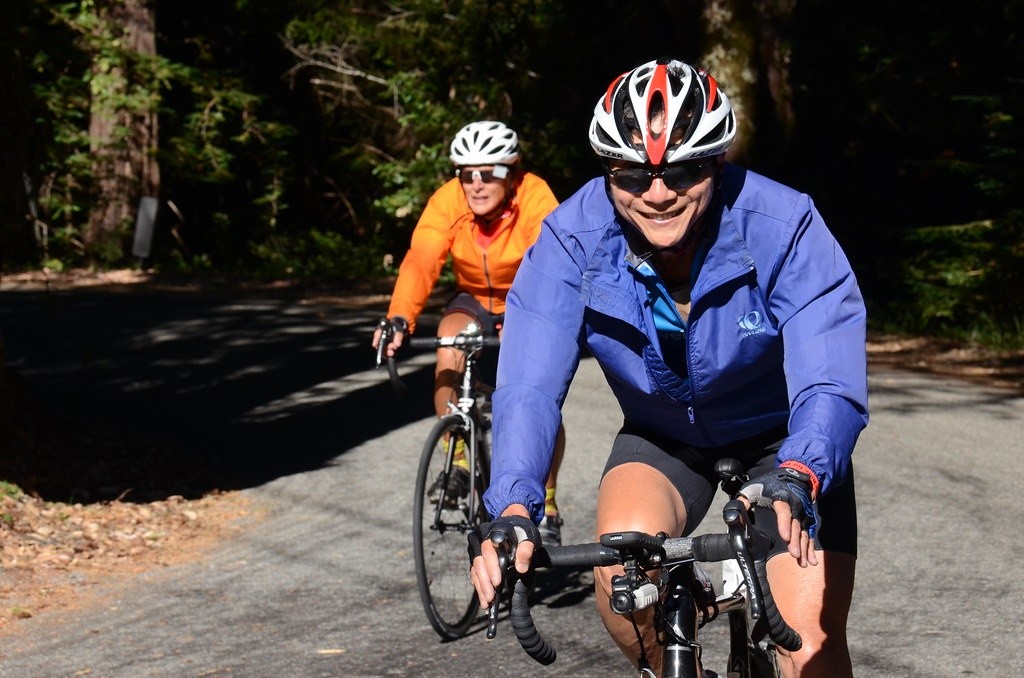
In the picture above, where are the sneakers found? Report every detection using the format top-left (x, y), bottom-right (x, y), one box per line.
top-left (537, 512), bottom-right (562, 548)
top-left (427, 463), bottom-right (471, 507)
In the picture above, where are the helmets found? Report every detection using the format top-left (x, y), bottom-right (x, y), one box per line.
top-left (588, 58), bottom-right (737, 167)
top-left (449, 121), bottom-right (520, 165)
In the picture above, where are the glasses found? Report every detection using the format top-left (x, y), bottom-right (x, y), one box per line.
top-left (454, 166), bottom-right (511, 184)
top-left (599, 156), bottom-right (718, 194)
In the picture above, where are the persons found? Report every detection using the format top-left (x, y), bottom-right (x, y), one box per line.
top-left (471, 60), bottom-right (895, 678)
top-left (369, 120), bottom-right (576, 548)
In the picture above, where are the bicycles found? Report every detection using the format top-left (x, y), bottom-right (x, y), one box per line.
top-left (486, 497), bottom-right (809, 678)
top-left (376, 313), bottom-right (527, 642)
